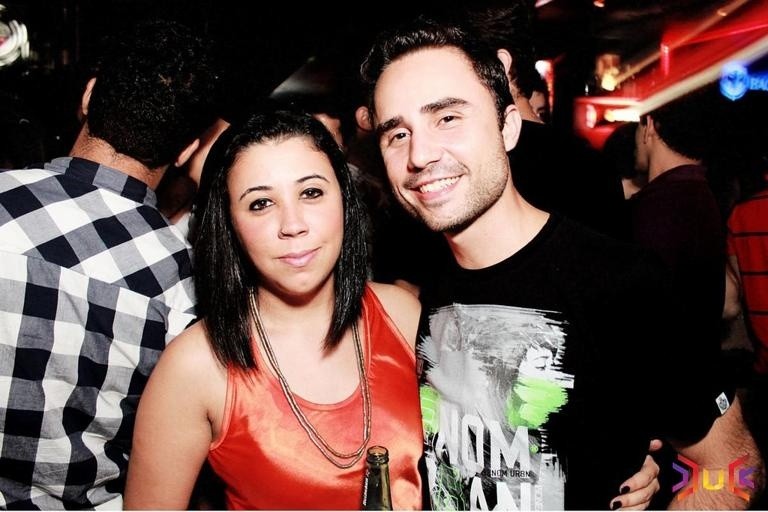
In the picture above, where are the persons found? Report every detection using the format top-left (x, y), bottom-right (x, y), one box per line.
top-left (0, 16), bottom-right (212, 511)
top-left (123, 110), bottom-right (422, 512)
top-left (245, 287), bottom-right (372, 470)
